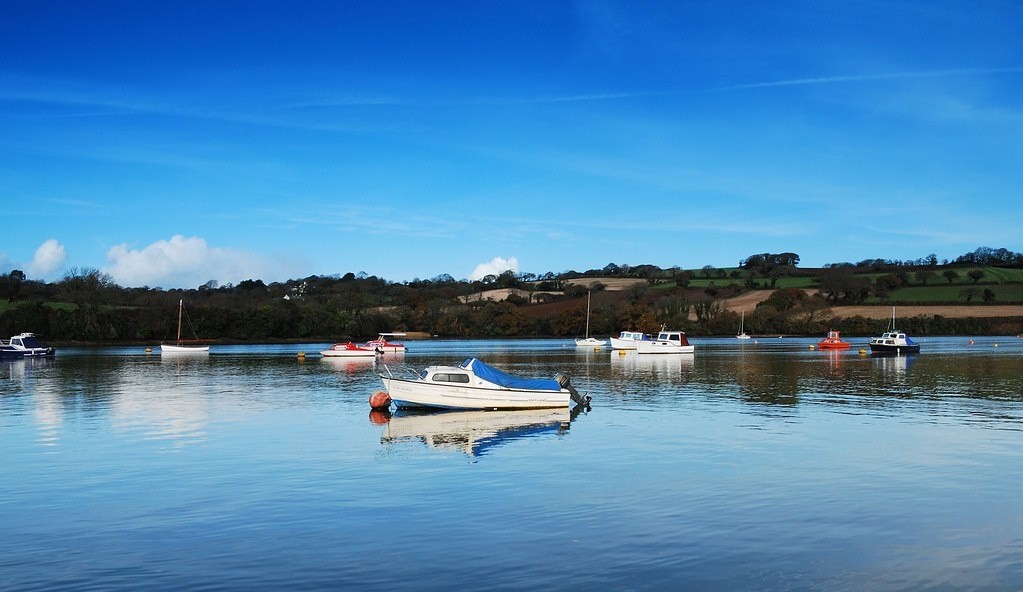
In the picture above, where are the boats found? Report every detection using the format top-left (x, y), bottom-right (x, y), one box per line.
top-left (610, 328), bottom-right (652, 350)
top-left (0, 333), bottom-right (56, 358)
top-left (817, 328), bottom-right (851, 349)
top-left (320, 335), bottom-right (409, 356)
top-left (637, 323), bottom-right (694, 354)
top-left (369, 356), bottom-right (592, 409)
top-left (868, 306), bottom-right (920, 353)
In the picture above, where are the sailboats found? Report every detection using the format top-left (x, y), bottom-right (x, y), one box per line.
top-left (735, 311), bottom-right (751, 339)
top-left (161, 299), bottom-right (210, 352)
top-left (575, 290), bottom-right (607, 346)
